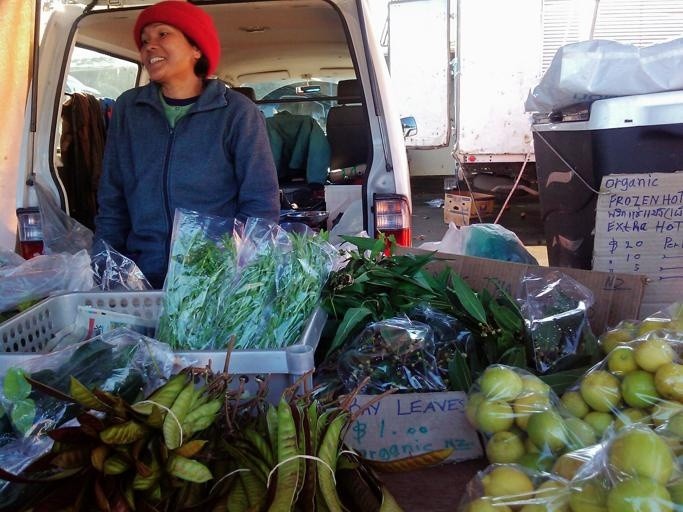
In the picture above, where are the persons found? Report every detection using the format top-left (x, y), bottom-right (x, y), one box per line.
top-left (86, 1), bottom-right (281, 293)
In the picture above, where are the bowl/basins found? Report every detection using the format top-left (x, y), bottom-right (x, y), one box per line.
top-left (280, 209), bottom-right (292, 221)
top-left (286, 210), bottom-right (329, 228)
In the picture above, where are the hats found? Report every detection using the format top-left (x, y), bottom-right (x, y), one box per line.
top-left (133, 1), bottom-right (220, 80)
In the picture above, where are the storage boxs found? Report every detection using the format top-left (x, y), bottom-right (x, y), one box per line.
top-left (443, 189), bottom-right (494, 227)
top-left (340, 244), bottom-right (648, 512)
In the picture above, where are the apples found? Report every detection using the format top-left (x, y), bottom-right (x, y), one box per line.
top-left (461, 309), bottom-right (683, 512)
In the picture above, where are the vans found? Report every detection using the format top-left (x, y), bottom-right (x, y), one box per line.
top-left (16, 0), bottom-right (418, 260)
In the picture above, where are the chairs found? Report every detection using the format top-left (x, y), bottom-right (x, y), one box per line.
top-left (229, 86), bottom-right (256, 105)
top-left (327, 78), bottom-right (372, 179)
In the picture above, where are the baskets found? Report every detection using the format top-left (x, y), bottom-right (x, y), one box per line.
top-left (1, 291), bottom-right (332, 414)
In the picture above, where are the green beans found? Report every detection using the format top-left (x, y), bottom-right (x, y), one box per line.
top-left (0, 360), bottom-right (453, 512)
